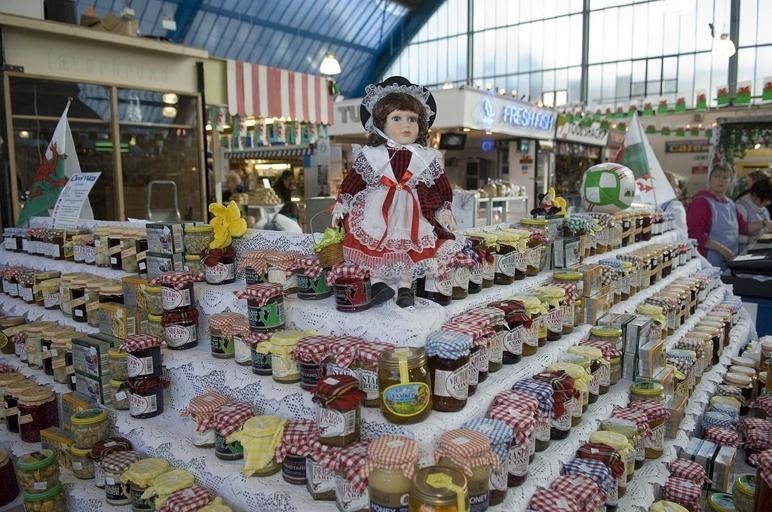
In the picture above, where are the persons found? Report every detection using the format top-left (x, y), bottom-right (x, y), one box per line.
top-left (735, 179), bottom-right (772, 254)
top-left (329, 75), bottom-right (481, 283)
top-left (222, 173), bottom-right (247, 202)
top-left (686, 163), bottom-right (772, 277)
top-left (273, 171), bottom-right (299, 219)
top-left (664, 169), bottom-right (683, 200)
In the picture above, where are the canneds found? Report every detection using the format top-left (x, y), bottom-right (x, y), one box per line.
top-left (0, 210), bottom-right (772, 512)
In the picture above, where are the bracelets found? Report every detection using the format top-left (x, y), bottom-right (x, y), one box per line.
top-left (763, 218), bottom-right (768, 226)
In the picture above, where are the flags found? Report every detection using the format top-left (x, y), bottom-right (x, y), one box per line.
top-left (16, 109), bottom-right (94, 228)
top-left (615, 109), bottom-right (677, 215)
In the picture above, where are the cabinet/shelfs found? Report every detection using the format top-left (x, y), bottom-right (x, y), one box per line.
top-left (1, 209), bottom-right (761, 511)
top-left (453, 188), bottom-right (529, 229)
